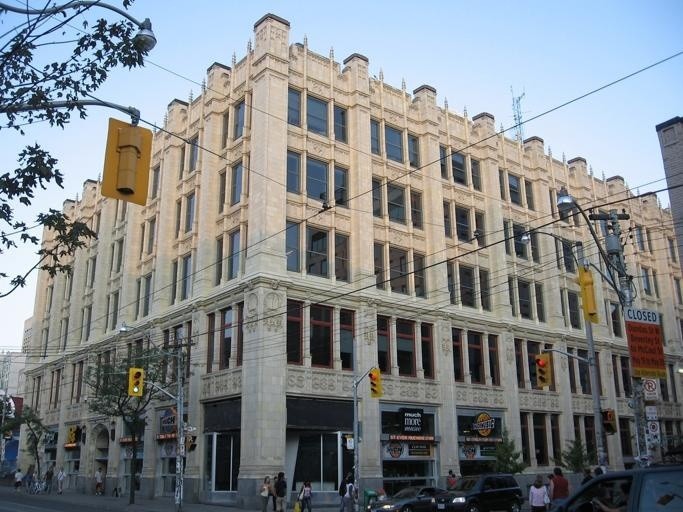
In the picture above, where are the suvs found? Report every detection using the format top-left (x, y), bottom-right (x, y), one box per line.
top-left (432, 473), bottom-right (523, 511)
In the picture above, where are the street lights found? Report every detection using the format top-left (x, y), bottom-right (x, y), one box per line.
top-left (520, 226), bottom-right (612, 472)
top-left (118, 321), bottom-right (183, 509)
top-left (556, 185), bottom-right (650, 471)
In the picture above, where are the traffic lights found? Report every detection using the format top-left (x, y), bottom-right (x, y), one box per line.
top-left (369, 368), bottom-right (383, 398)
top-left (127, 366), bottom-right (143, 396)
top-left (533, 350), bottom-right (552, 386)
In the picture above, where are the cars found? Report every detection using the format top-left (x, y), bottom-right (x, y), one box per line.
top-left (366, 483), bottom-right (444, 512)
top-left (548, 468), bottom-right (682, 512)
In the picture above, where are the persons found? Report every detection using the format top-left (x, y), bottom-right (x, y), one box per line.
top-left (95, 467), bottom-right (104, 496)
top-left (339, 470), bottom-right (355, 511)
top-left (343, 475), bottom-right (357, 512)
top-left (14, 466), bottom-right (67, 495)
top-left (447, 470), bottom-right (453, 490)
top-left (448, 474), bottom-right (457, 491)
top-left (528, 468), bottom-right (630, 512)
top-left (259, 471), bottom-right (313, 512)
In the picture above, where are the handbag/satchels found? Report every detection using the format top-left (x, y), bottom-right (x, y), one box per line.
top-left (543, 494), bottom-right (550, 504)
top-left (296, 491), bottom-right (303, 501)
